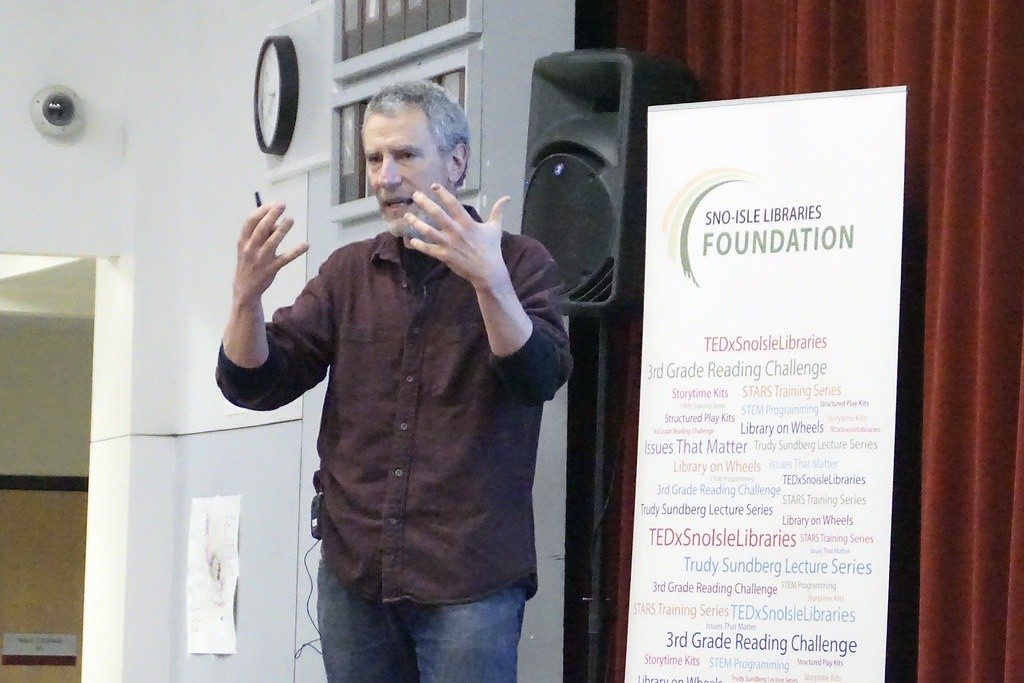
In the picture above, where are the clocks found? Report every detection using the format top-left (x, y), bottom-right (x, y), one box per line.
top-left (252, 34), bottom-right (298, 156)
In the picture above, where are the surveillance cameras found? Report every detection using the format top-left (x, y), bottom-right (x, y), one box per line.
top-left (30, 85), bottom-right (84, 137)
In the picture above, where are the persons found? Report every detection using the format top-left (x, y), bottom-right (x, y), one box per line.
top-left (215, 80), bottom-right (574, 682)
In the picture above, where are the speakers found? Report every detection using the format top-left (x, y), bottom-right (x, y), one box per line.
top-left (521, 46), bottom-right (702, 324)
